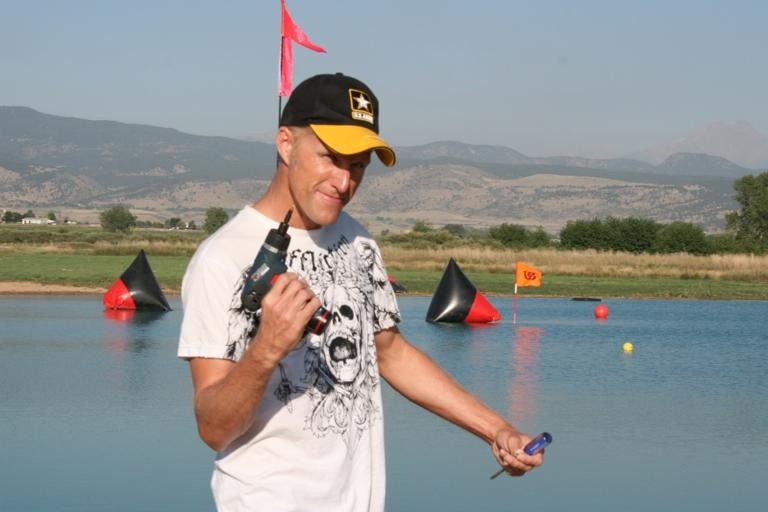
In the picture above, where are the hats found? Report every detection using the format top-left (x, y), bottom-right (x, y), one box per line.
top-left (279, 72), bottom-right (396, 168)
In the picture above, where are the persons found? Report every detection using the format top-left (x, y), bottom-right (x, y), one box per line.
top-left (175, 71), bottom-right (545, 512)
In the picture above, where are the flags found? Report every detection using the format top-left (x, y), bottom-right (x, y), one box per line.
top-left (515, 260), bottom-right (542, 288)
top-left (279, 0), bottom-right (328, 96)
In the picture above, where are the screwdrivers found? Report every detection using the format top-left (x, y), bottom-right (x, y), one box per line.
top-left (491, 432), bottom-right (552, 480)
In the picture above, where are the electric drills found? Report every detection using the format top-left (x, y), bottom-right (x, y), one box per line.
top-left (241, 207), bottom-right (332, 331)
